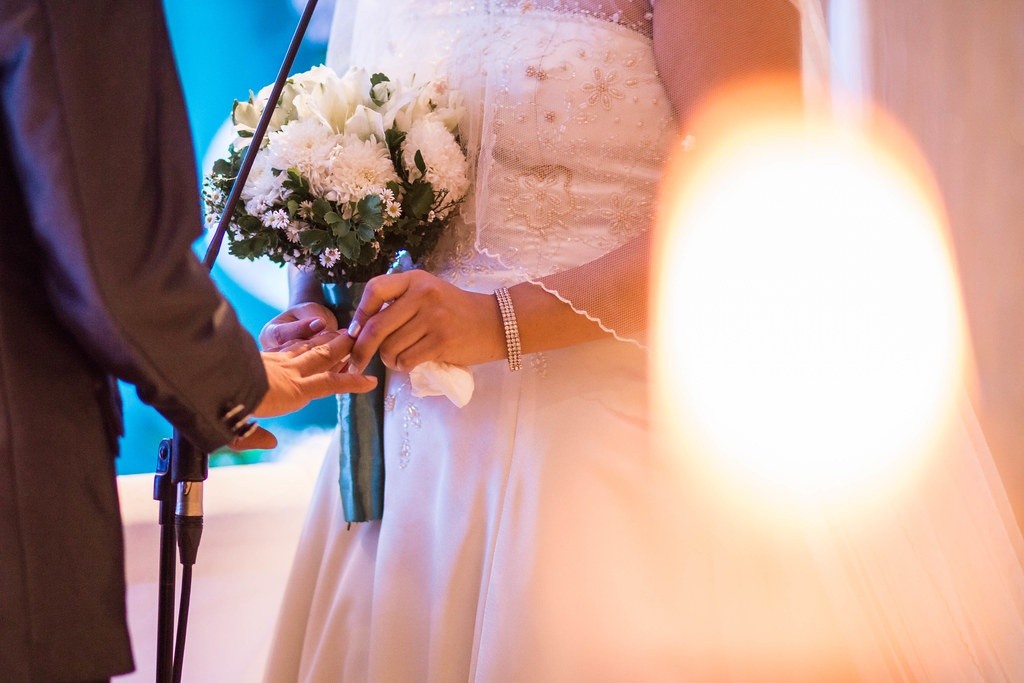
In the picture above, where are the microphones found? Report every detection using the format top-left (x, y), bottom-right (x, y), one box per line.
top-left (175, 422), bottom-right (209, 566)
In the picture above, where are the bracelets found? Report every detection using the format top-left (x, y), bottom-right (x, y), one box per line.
top-left (494, 289), bottom-right (527, 377)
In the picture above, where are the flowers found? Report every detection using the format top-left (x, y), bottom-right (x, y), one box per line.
top-left (200, 61), bottom-right (474, 522)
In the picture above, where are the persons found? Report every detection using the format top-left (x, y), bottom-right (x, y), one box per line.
top-left (0, 0), bottom-right (378, 683)
top-left (252, 0), bottom-right (1024, 683)
